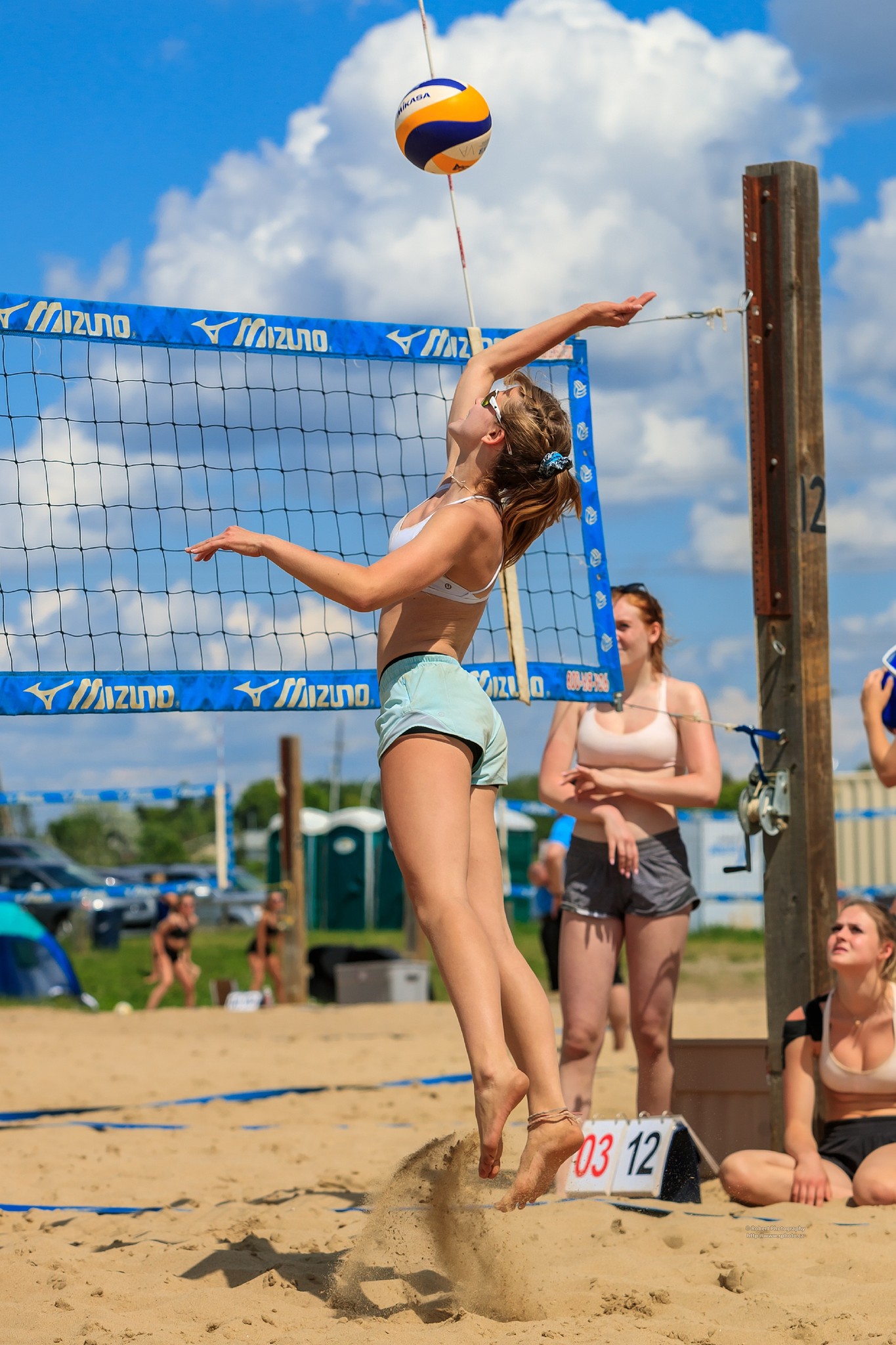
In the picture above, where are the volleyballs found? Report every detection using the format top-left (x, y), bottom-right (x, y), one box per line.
top-left (113, 1001), bottom-right (133, 1015)
top-left (394, 78), bottom-right (493, 175)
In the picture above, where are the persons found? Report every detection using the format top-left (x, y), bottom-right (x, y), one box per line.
top-left (186, 289), bottom-right (660, 1210)
top-left (138, 874), bottom-right (295, 1009)
top-left (522, 584), bottom-right (896, 1208)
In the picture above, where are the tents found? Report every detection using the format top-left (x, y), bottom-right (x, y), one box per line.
top-left (1, 901), bottom-right (82, 1002)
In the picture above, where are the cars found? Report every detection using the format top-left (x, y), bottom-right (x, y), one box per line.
top-left (0, 835), bottom-right (267, 937)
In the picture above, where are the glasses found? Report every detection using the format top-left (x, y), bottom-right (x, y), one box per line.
top-left (481, 386), bottom-right (519, 456)
top-left (611, 583), bottom-right (657, 618)
